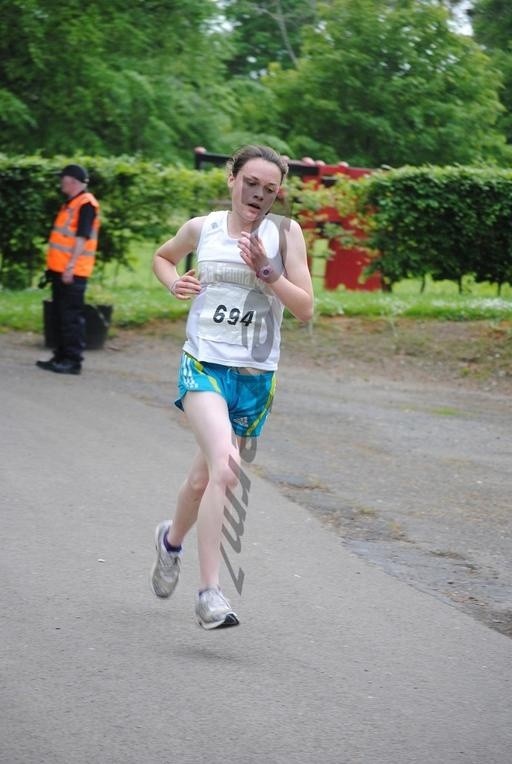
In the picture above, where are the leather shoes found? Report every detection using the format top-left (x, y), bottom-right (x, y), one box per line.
top-left (36, 360), bottom-right (81, 375)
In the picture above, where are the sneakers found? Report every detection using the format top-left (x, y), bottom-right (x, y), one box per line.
top-left (194, 591), bottom-right (240, 630)
top-left (151, 521), bottom-right (180, 597)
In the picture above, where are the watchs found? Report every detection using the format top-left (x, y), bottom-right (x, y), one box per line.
top-left (256, 262), bottom-right (273, 279)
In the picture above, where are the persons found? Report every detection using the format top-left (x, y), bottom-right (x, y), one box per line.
top-left (35, 164), bottom-right (101, 375)
top-left (148, 144), bottom-right (319, 631)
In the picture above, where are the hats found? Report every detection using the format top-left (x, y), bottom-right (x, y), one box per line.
top-left (55, 165), bottom-right (88, 183)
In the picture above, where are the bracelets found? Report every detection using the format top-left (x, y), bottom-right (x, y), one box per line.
top-left (170, 276), bottom-right (180, 294)
top-left (66, 264), bottom-right (75, 272)
